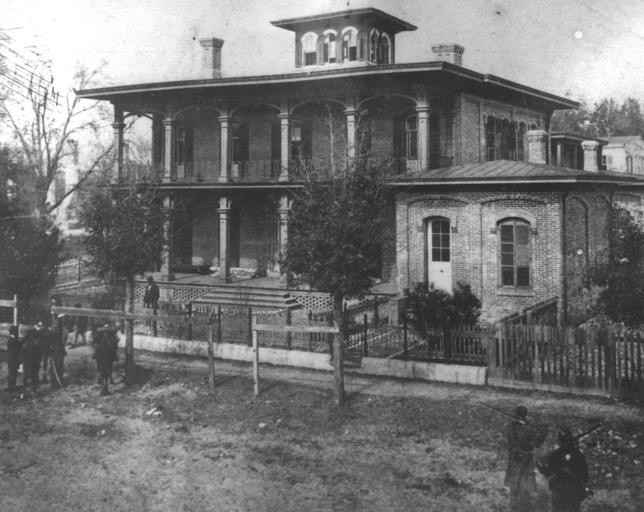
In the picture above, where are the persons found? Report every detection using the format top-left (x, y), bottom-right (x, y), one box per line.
top-left (92, 325), bottom-right (118, 392)
top-left (141, 275), bottom-right (158, 335)
top-left (535, 427), bottom-right (589, 511)
top-left (506, 406), bottom-right (548, 512)
top-left (5, 303), bottom-right (85, 390)
